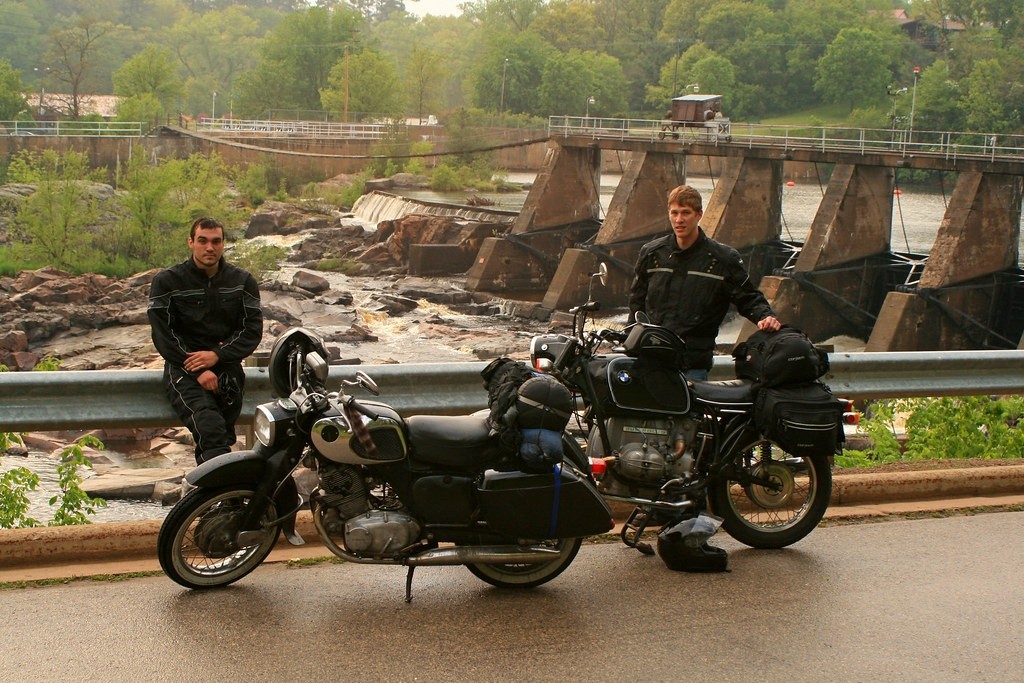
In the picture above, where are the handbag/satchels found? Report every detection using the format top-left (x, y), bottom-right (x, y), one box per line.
top-left (731, 323), bottom-right (845, 457)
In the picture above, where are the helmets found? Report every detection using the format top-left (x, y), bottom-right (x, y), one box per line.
top-left (268, 326), bottom-right (329, 397)
top-left (657, 516), bottom-right (728, 572)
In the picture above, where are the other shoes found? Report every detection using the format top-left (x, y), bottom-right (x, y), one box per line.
top-left (632, 514), bottom-right (661, 527)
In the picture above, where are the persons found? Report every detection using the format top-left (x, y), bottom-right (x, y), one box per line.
top-left (626, 185), bottom-right (782, 527)
top-left (146, 217), bottom-right (263, 531)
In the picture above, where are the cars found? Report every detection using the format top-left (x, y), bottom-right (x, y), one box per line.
top-left (10, 131), bottom-right (35, 136)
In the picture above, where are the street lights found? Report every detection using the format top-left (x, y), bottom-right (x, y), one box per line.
top-left (499, 58), bottom-right (511, 115)
top-left (212, 90), bottom-right (217, 128)
top-left (908, 66), bottom-right (920, 144)
top-left (585, 96), bottom-right (596, 133)
top-left (683, 83), bottom-right (701, 95)
top-left (32, 66), bottom-right (50, 128)
top-left (885, 85), bottom-right (907, 150)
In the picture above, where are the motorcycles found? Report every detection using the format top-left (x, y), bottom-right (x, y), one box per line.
top-left (154, 338), bottom-right (617, 606)
top-left (529, 260), bottom-right (862, 555)
